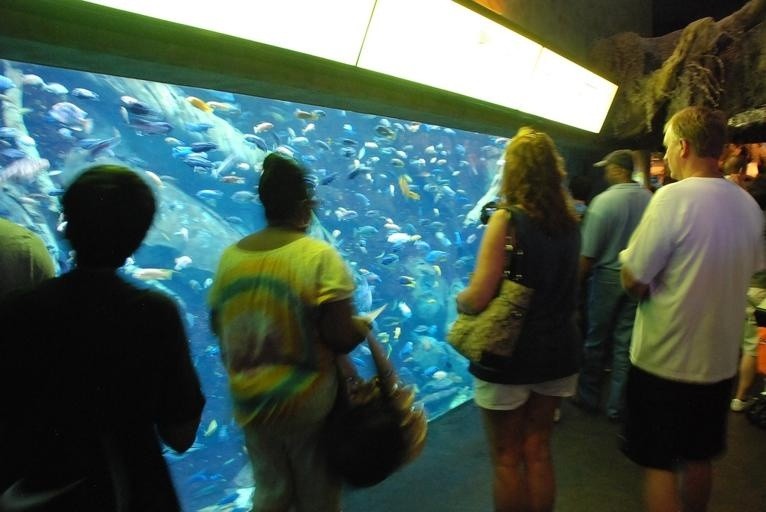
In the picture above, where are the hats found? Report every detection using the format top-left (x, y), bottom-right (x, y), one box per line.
top-left (594, 148), bottom-right (635, 171)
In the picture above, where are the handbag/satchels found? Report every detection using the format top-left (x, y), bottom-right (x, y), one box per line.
top-left (445, 203), bottom-right (536, 373)
top-left (322, 316), bottom-right (429, 488)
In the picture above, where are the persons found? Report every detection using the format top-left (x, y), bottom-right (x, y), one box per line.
top-left (618, 107), bottom-right (765, 509)
top-left (0, 217), bottom-right (59, 509)
top-left (208, 153), bottom-right (371, 510)
top-left (22, 162), bottom-right (205, 509)
top-left (457, 129), bottom-right (581, 509)
top-left (644, 138), bottom-right (675, 192)
top-left (724, 112), bottom-right (766, 411)
top-left (571, 151), bottom-right (654, 424)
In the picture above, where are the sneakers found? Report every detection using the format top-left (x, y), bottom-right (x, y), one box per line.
top-left (598, 405), bottom-right (619, 419)
top-left (729, 397), bottom-right (759, 412)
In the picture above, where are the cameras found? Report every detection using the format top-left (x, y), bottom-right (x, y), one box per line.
top-left (478, 200), bottom-right (503, 226)
